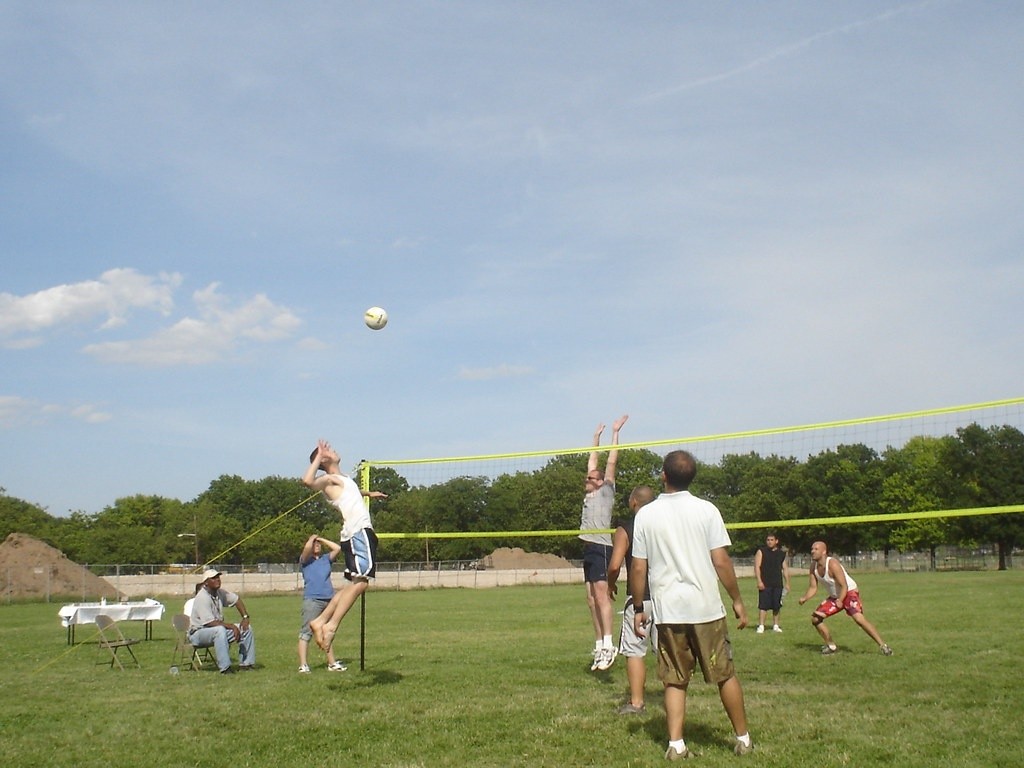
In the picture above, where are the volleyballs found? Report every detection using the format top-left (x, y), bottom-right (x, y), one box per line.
top-left (365, 306), bottom-right (388, 331)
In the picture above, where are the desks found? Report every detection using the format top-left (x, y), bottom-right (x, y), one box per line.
top-left (57, 598), bottom-right (165, 646)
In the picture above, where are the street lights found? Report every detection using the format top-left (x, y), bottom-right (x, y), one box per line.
top-left (176, 533), bottom-right (199, 570)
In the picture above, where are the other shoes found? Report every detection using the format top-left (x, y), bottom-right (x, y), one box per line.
top-left (757, 624), bottom-right (764, 633)
top-left (221, 666), bottom-right (236, 676)
top-left (821, 644), bottom-right (838, 656)
top-left (773, 624), bottom-right (783, 632)
top-left (238, 666), bottom-right (255, 671)
top-left (880, 643), bottom-right (893, 656)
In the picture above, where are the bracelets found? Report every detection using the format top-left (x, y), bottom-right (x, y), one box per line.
top-left (633, 604), bottom-right (644, 614)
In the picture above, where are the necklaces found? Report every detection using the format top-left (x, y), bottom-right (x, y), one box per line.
top-left (212, 597), bottom-right (219, 612)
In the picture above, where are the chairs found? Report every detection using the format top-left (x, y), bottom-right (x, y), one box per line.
top-left (94, 614), bottom-right (142, 672)
top-left (171, 615), bottom-right (219, 675)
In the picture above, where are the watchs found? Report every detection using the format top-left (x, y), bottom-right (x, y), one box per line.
top-left (243, 615), bottom-right (249, 618)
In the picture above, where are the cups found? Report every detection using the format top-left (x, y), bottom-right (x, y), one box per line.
top-left (100, 596), bottom-right (107, 606)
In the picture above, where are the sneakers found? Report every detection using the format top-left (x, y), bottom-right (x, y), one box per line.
top-left (299, 664), bottom-right (311, 674)
top-left (591, 648), bottom-right (603, 671)
top-left (664, 744), bottom-right (703, 763)
top-left (616, 701), bottom-right (647, 716)
top-left (733, 741), bottom-right (754, 757)
top-left (598, 646), bottom-right (618, 671)
top-left (328, 661), bottom-right (347, 672)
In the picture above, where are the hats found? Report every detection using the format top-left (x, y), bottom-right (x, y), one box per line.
top-left (202, 569), bottom-right (222, 583)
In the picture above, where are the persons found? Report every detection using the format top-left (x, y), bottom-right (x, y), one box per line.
top-left (607, 485), bottom-right (658, 713)
top-left (578, 416), bottom-right (629, 670)
top-left (799, 542), bottom-right (893, 657)
top-left (299, 535), bottom-right (349, 673)
top-left (754, 534), bottom-right (790, 634)
top-left (304, 437), bottom-right (388, 653)
top-left (629, 451), bottom-right (752, 760)
top-left (184, 569), bottom-right (256, 674)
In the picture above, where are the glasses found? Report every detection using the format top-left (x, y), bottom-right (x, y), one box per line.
top-left (588, 476), bottom-right (598, 480)
